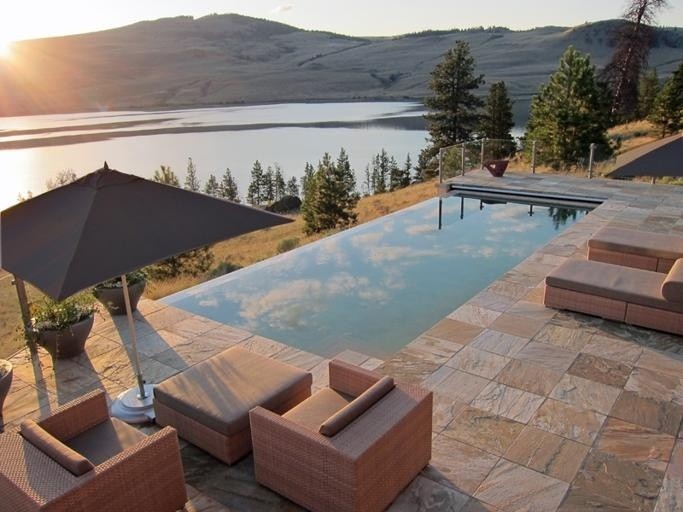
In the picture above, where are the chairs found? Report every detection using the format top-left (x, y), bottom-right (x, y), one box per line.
top-left (1, 388), bottom-right (188, 512)
top-left (248, 359), bottom-right (433, 511)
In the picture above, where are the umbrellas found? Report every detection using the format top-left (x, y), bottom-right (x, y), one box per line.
top-left (0, 162), bottom-right (296, 395)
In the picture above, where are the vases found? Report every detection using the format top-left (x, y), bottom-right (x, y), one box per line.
top-left (0, 357), bottom-right (12, 431)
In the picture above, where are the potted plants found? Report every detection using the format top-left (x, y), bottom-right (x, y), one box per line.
top-left (93, 268), bottom-right (148, 315)
top-left (15, 294), bottom-right (114, 380)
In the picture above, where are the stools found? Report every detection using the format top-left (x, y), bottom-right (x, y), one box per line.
top-left (153, 346), bottom-right (312, 463)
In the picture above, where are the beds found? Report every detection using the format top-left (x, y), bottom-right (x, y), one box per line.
top-left (544, 258), bottom-right (683, 336)
top-left (587, 226), bottom-right (683, 273)
